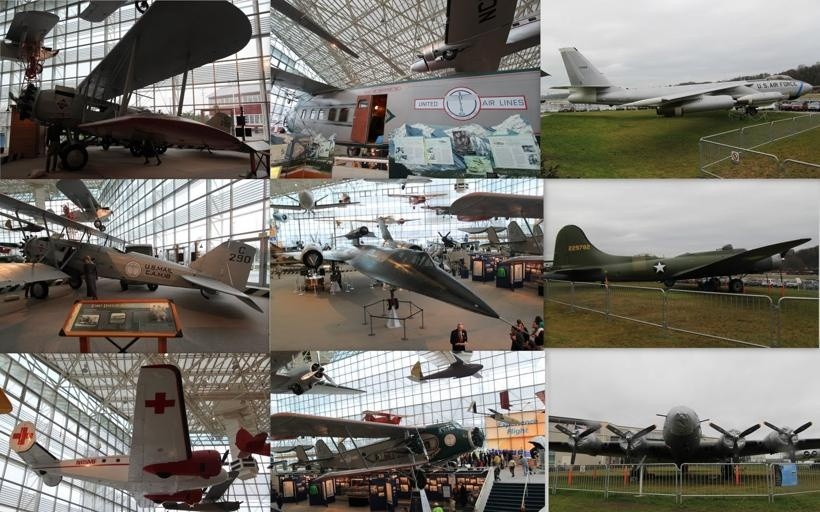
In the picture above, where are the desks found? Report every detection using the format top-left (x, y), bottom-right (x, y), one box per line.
top-left (59, 298), bottom-right (183, 352)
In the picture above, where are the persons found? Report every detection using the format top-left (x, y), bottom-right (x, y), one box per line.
top-left (46, 126), bottom-right (61, 174)
top-left (450, 321), bottom-right (468, 350)
top-left (275, 493), bottom-right (283, 509)
top-left (142, 135), bottom-right (162, 166)
top-left (535, 315), bottom-right (544, 332)
top-left (600, 271), bottom-right (606, 289)
top-left (432, 502), bottom-right (445, 512)
top-left (446, 497), bottom-right (457, 511)
top-left (509, 326), bottom-right (526, 351)
top-left (459, 449), bottom-right (538, 483)
top-left (517, 318), bottom-right (530, 343)
top-left (531, 320), bottom-right (544, 350)
top-left (84, 255), bottom-right (99, 301)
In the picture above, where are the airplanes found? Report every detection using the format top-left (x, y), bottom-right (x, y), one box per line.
top-left (0, 0), bottom-right (260, 172)
top-left (547, 404), bottom-right (820, 483)
top-left (0, 179), bottom-right (271, 313)
top-left (545, 224), bottom-right (812, 294)
top-left (545, 46), bottom-right (813, 119)
top-left (270, 352), bottom-right (527, 486)
top-left (271, 177), bottom-right (545, 341)
top-left (8, 362), bottom-right (241, 512)
top-left (270, 0), bottom-right (540, 173)
